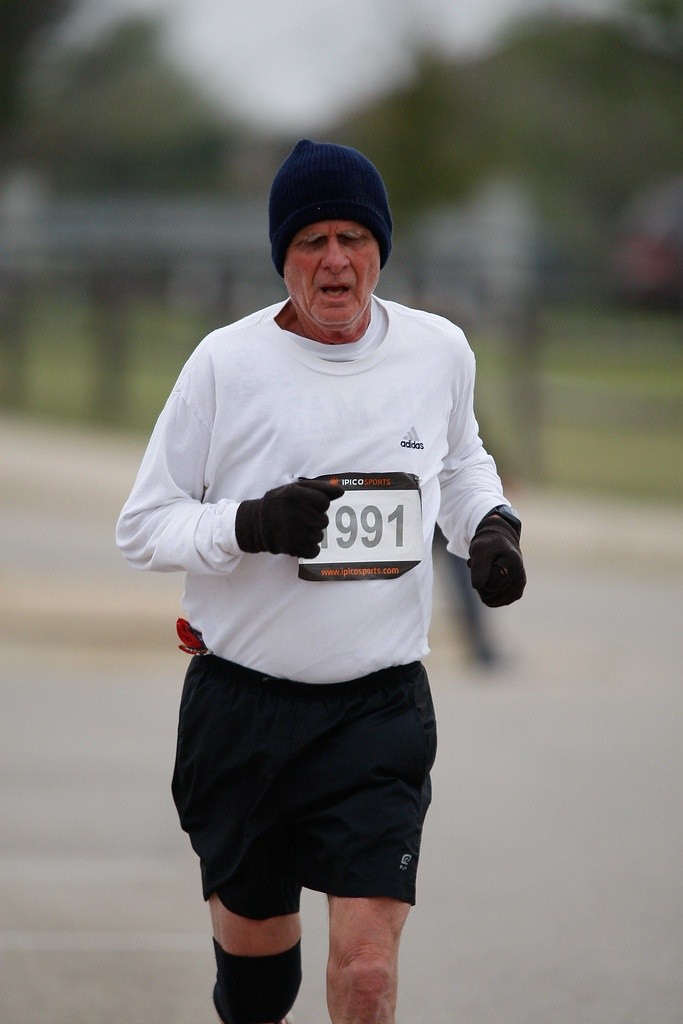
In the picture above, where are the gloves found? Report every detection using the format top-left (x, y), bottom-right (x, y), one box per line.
top-left (235, 481), bottom-right (344, 559)
top-left (466, 515), bottom-right (526, 609)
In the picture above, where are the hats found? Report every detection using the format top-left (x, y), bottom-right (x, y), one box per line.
top-left (268, 139), bottom-right (392, 280)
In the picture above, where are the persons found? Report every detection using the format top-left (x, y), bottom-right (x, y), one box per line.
top-left (114, 141), bottom-right (531, 1021)
